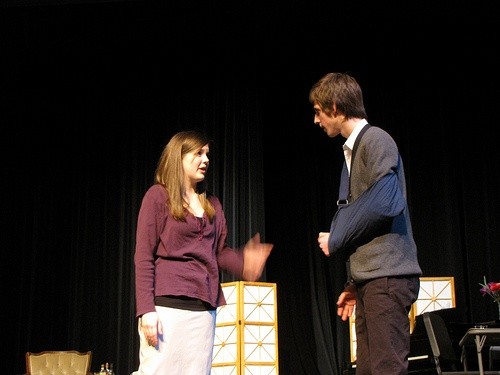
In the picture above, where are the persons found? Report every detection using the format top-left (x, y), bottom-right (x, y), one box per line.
top-left (310, 72), bottom-right (422, 375)
top-left (135, 129), bottom-right (274, 375)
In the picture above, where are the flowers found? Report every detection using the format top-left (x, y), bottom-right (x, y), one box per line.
top-left (479, 276), bottom-right (500, 325)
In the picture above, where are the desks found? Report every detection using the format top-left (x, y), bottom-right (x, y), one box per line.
top-left (458, 328), bottom-right (500, 375)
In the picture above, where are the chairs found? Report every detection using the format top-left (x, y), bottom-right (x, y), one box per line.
top-left (25, 350), bottom-right (93, 375)
top-left (423, 312), bottom-right (480, 375)
